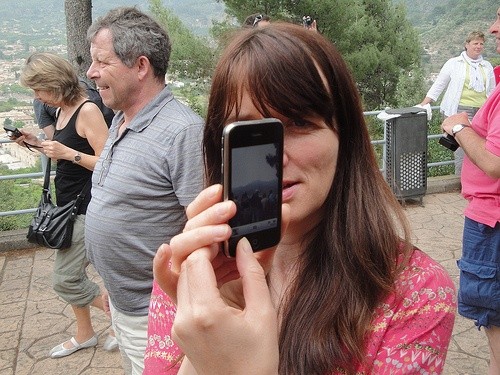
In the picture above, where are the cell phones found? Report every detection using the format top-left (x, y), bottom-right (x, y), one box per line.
top-left (223, 119), bottom-right (284, 257)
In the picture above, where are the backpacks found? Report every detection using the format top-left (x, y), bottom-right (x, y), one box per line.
top-left (79, 78), bottom-right (114, 130)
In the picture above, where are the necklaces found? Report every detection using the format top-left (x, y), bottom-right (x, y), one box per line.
top-left (58, 105), bottom-right (76, 127)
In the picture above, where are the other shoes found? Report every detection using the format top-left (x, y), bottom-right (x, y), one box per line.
top-left (103, 327), bottom-right (117, 352)
top-left (49, 333), bottom-right (97, 358)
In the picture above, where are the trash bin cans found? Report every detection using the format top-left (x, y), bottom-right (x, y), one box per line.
top-left (384, 107), bottom-right (428, 208)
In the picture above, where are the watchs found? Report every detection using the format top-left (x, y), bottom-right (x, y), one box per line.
top-left (451, 124), bottom-right (470, 137)
top-left (72, 151), bottom-right (82, 164)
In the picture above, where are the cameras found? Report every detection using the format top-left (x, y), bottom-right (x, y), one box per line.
top-left (3, 125), bottom-right (22, 138)
top-left (439, 133), bottom-right (459, 151)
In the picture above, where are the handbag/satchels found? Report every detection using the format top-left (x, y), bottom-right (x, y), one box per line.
top-left (28, 193), bottom-right (76, 250)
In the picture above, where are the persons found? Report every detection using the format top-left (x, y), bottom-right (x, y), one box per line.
top-left (8, 49), bottom-right (119, 359)
top-left (238, 189), bottom-right (277, 220)
top-left (29, 80), bottom-right (115, 140)
top-left (242, 11), bottom-right (318, 34)
top-left (89, 3), bottom-right (207, 375)
top-left (440, 5), bottom-right (500, 375)
top-left (422, 30), bottom-right (496, 175)
top-left (140, 20), bottom-right (458, 375)
